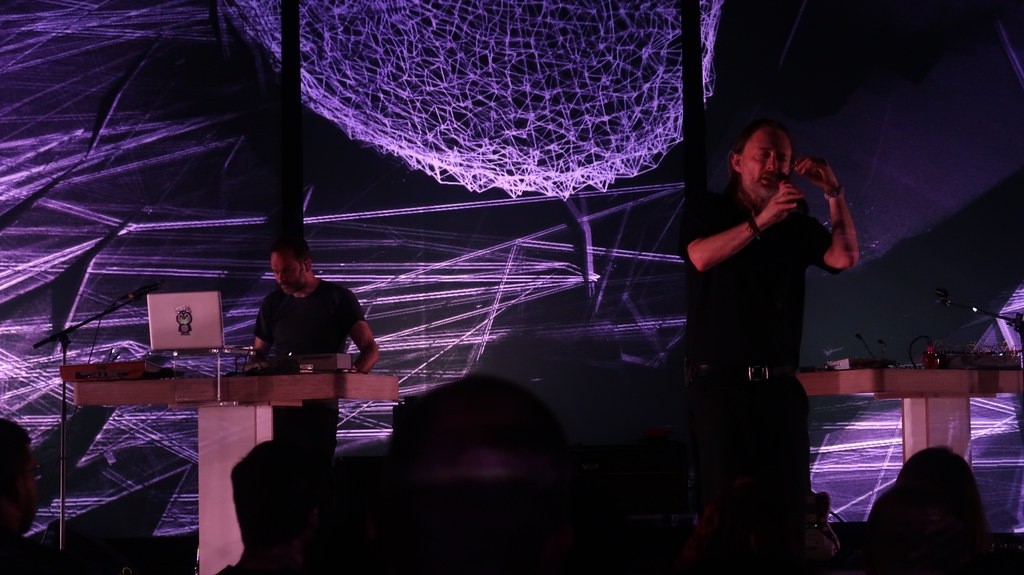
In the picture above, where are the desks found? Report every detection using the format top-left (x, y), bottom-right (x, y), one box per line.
top-left (797, 368), bottom-right (1024, 470)
top-left (73, 372), bottom-right (398, 575)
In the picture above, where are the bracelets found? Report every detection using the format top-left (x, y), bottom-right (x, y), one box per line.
top-left (747, 216), bottom-right (761, 240)
top-left (824, 184), bottom-right (847, 200)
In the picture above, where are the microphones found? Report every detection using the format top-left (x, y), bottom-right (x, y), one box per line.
top-left (122, 279), bottom-right (168, 301)
top-left (769, 173), bottom-right (811, 215)
top-left (935, 288), bottom-right (952, 299)
top-left (855, 334), bottom-right (876, 359)
top-left (878, 339), bottom-right (887, 360)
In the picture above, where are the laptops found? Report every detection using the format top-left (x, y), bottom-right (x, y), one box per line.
top-left (145, 291), bottom-right (254, 350)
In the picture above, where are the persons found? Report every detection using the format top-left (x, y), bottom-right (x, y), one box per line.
top-left (677, 118), bottom-right (860, 493)
top-left (247, 233), bottom-right (380, 461)
top-left (217, 375), bottom-right (1024, 575)
top-left (0, 419), bottom-right (142, 575)
